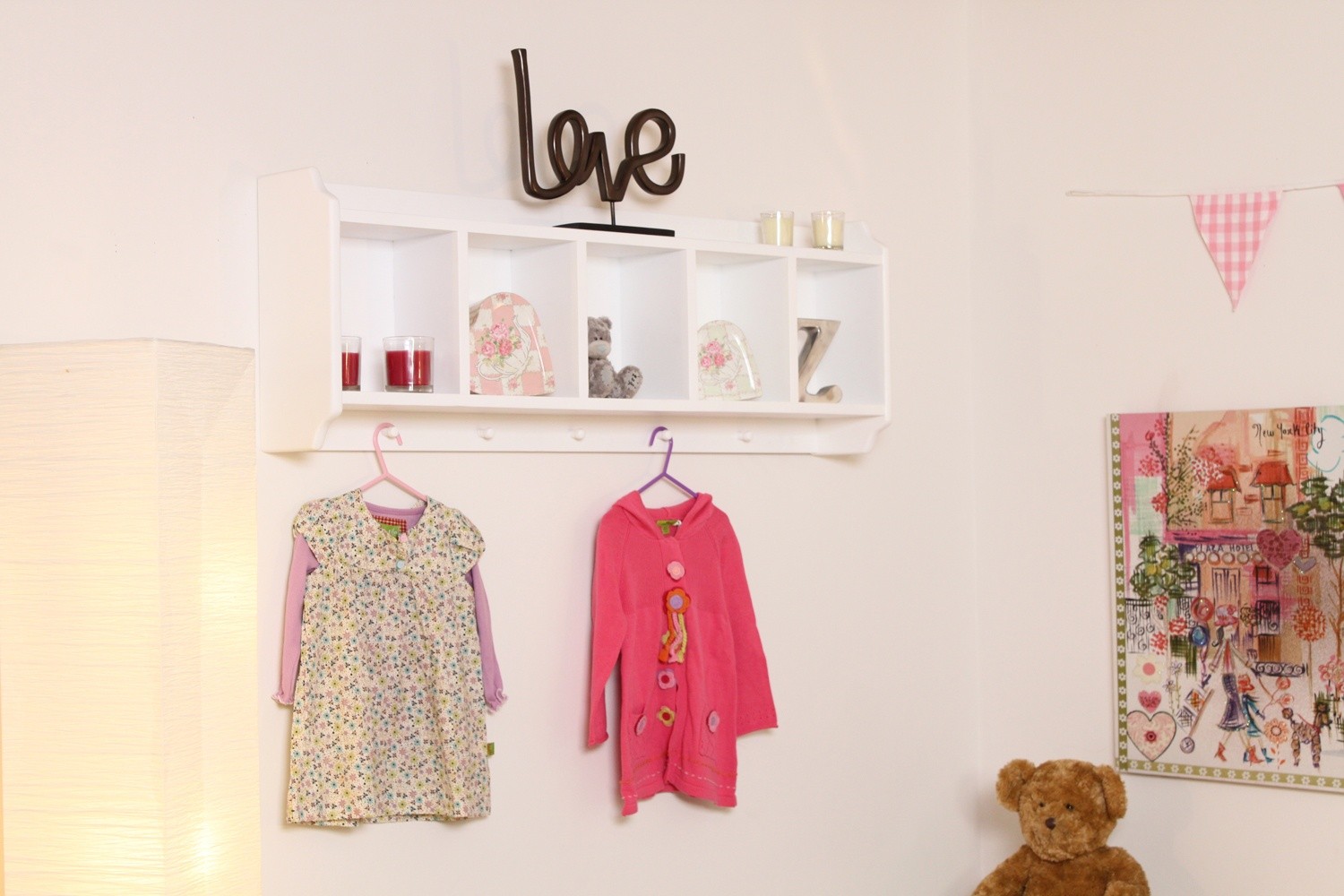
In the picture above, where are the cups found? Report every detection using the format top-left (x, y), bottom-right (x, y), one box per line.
top-left (383, 336), bottom-right (434, 392)
top-left (342, 334), bottom-right (363, 389)
top-left (812, 211), bottom-right (845, 249)
top-left (760, 209), bottom-right (795, 247)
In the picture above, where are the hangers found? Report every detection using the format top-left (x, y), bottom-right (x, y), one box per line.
top-left (638, 425), bottom-right (695, 498)
top-left (360, 422), bottom-right (428, 503)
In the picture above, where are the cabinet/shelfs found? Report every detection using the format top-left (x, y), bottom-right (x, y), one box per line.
top-left (252, 168), bottom-right (890, 456)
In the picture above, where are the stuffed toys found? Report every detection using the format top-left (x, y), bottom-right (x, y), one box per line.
top-left (588, 316), bottom-right (643, 400)
top-left (970, 758), bottom-right (1151, 896)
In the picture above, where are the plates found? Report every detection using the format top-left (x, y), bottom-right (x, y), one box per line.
top-left (696, 319), bottom-right (764, 400)
top-left (468, 291), bottom-right (559, 395)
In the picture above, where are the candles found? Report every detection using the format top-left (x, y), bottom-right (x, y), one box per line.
top-left (811, 209), bottom-right (846, 250)
top-left (381, 335), bottom-right (434, 393)
top-left (342, 335), bottom-right (362, 391)
top-left (760, 209), bottom-right (795, 248)
